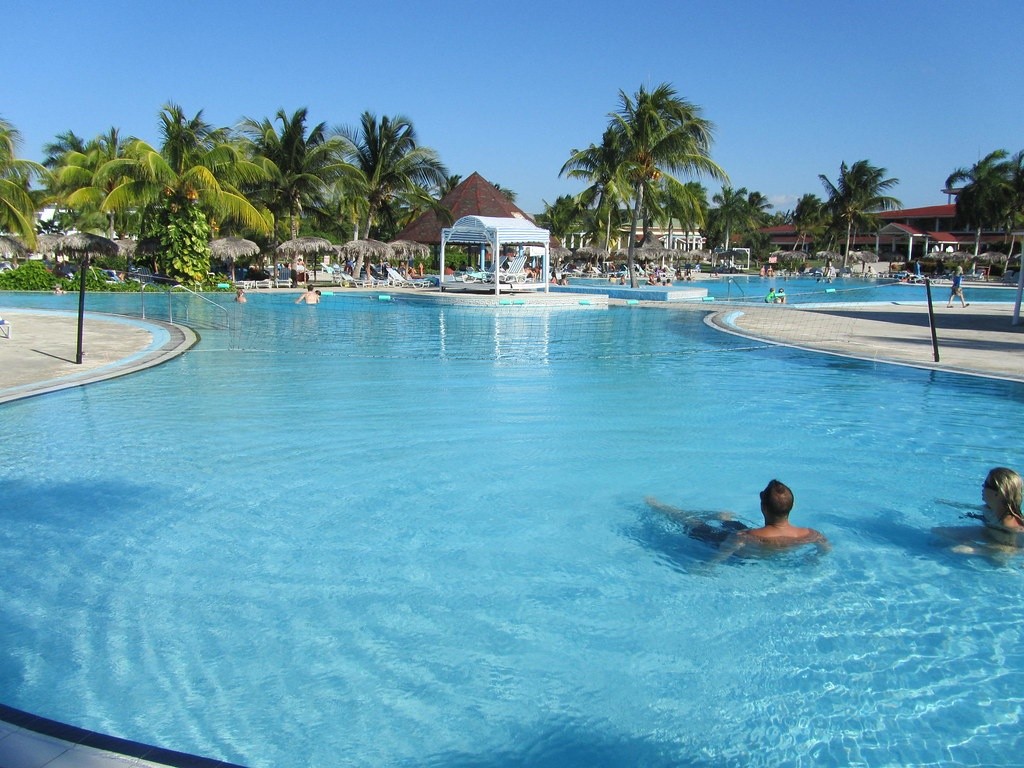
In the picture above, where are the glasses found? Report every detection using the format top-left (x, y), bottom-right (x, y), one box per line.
top-left (981, 480), bottom-right (995, 491)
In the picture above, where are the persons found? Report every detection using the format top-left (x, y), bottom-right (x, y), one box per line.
top-left (931, 467), bottom-right (1023, 559)
top-left (644, 478), bottom-right (832, 579)
top-left (0, 242), bottom-right (1011, 309)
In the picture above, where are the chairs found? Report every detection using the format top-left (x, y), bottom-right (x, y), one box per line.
top-left (89, 255), bottom-right (1020, 289)
top-left (0, 315), bottom-right (10, 339)
